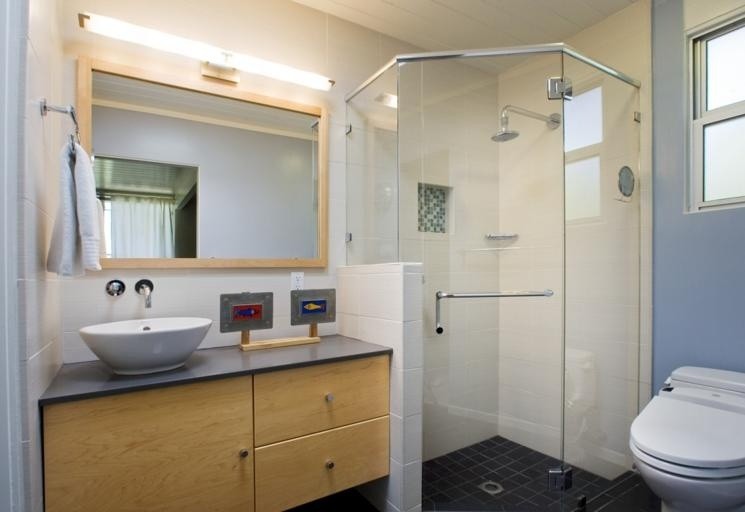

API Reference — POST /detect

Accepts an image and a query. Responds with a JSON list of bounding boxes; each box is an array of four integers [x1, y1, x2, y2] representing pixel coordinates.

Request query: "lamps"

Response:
[[73, 9, 337, 95]]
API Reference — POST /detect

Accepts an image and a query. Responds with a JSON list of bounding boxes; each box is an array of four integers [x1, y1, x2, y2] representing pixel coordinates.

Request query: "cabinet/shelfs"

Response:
[[251, 351, 396, 512], [41, 372, 254, 510]]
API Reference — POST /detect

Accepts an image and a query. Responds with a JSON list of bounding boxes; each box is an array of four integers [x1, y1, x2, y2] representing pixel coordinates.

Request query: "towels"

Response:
[[41, 137, 104, 280]]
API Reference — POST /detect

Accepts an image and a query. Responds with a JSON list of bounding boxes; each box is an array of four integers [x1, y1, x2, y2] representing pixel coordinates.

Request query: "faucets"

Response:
[[136, 279, 154, 308]]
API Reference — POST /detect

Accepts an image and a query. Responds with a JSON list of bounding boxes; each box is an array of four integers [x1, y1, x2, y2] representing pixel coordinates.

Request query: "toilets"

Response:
[[628, 365, 745, 512]]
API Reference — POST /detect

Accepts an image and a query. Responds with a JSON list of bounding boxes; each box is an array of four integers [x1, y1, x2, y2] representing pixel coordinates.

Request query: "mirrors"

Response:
[[69, 49, 335, 276]]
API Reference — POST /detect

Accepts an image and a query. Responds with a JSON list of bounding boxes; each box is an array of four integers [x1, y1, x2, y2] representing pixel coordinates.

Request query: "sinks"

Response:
[[79, 317, 213, 375]]
